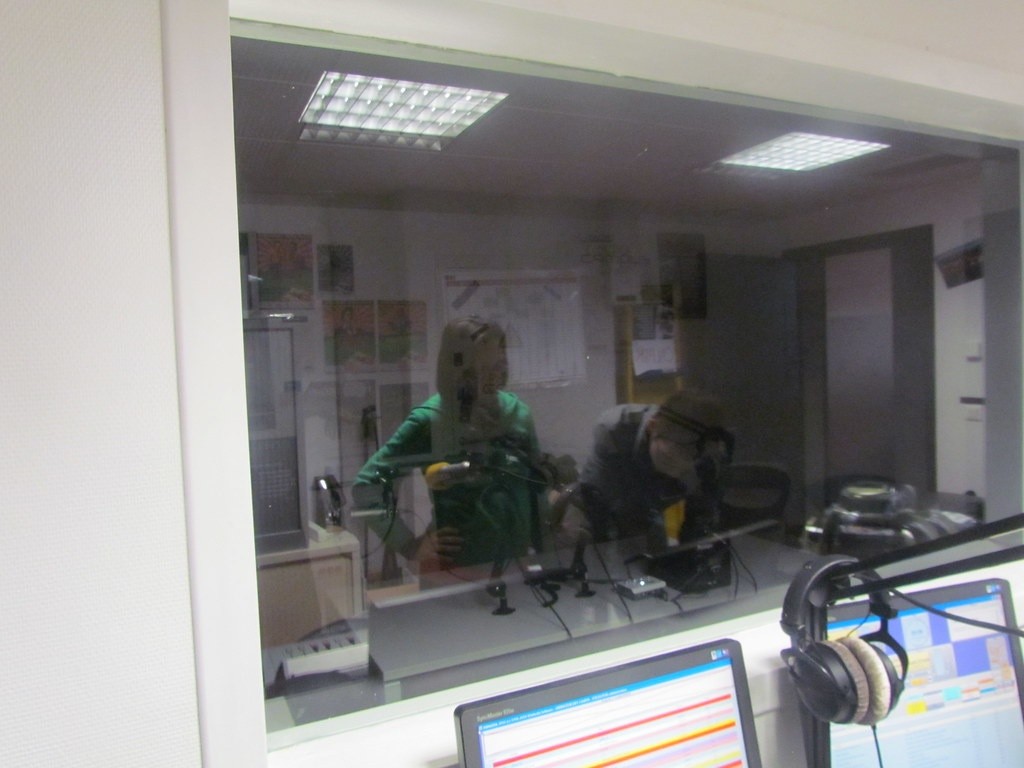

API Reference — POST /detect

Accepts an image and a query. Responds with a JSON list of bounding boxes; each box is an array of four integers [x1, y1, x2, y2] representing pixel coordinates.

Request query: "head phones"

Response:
[[780, 554, 908, 726]]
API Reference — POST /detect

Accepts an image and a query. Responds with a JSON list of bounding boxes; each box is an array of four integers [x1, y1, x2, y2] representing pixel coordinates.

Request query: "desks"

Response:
[[361, 528, 818, 682]]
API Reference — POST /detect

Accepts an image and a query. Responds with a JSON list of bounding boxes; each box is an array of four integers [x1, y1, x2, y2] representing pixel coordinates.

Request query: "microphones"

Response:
[[426, 462, 484, 490]]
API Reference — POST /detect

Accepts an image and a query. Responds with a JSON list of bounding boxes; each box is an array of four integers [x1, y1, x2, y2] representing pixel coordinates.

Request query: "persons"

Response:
[[561, 404, 724, 551], [351, 319, 555, 595]]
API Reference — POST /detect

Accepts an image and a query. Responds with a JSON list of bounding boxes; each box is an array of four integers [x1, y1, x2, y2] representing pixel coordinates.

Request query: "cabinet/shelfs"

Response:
[[259, 521, 364, 647]]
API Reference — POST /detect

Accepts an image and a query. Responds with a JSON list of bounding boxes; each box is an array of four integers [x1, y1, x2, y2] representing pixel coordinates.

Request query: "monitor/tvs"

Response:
[[453, 638, 763, 768], [813, 576, 1024, 768]]
